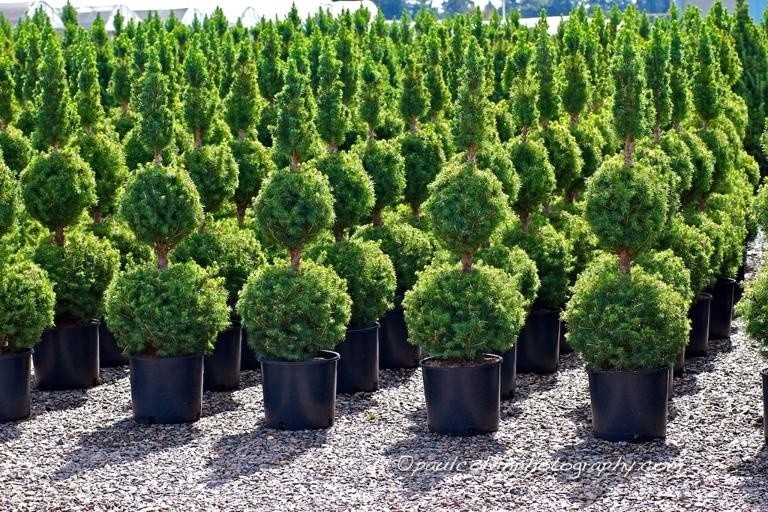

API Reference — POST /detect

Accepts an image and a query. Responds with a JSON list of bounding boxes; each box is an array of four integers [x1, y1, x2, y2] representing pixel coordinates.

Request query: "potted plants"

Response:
[[0, 0, 768, 445]]
